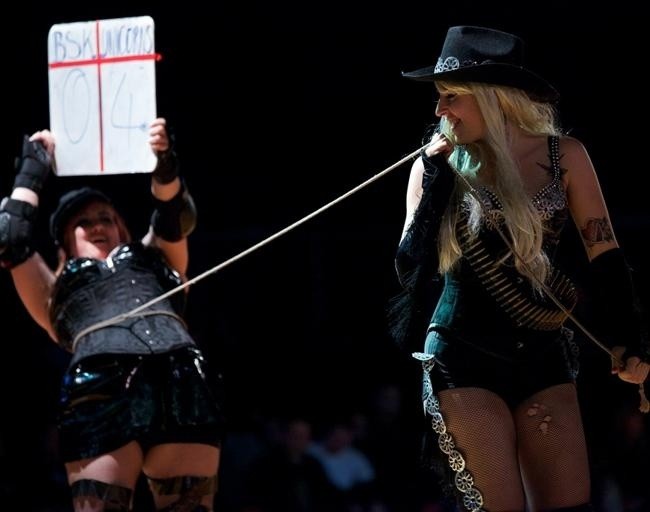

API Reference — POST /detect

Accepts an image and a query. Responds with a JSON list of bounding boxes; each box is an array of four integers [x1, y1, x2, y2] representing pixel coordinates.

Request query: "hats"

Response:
[[49, 187, 111, 244], [403, 26, 560, 103]]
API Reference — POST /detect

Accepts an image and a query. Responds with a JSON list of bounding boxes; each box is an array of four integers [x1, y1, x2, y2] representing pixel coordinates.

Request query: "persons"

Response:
[[395, 26, 649, 512], [1, 118, 222, 512], [247, 388, 441, 511]]
